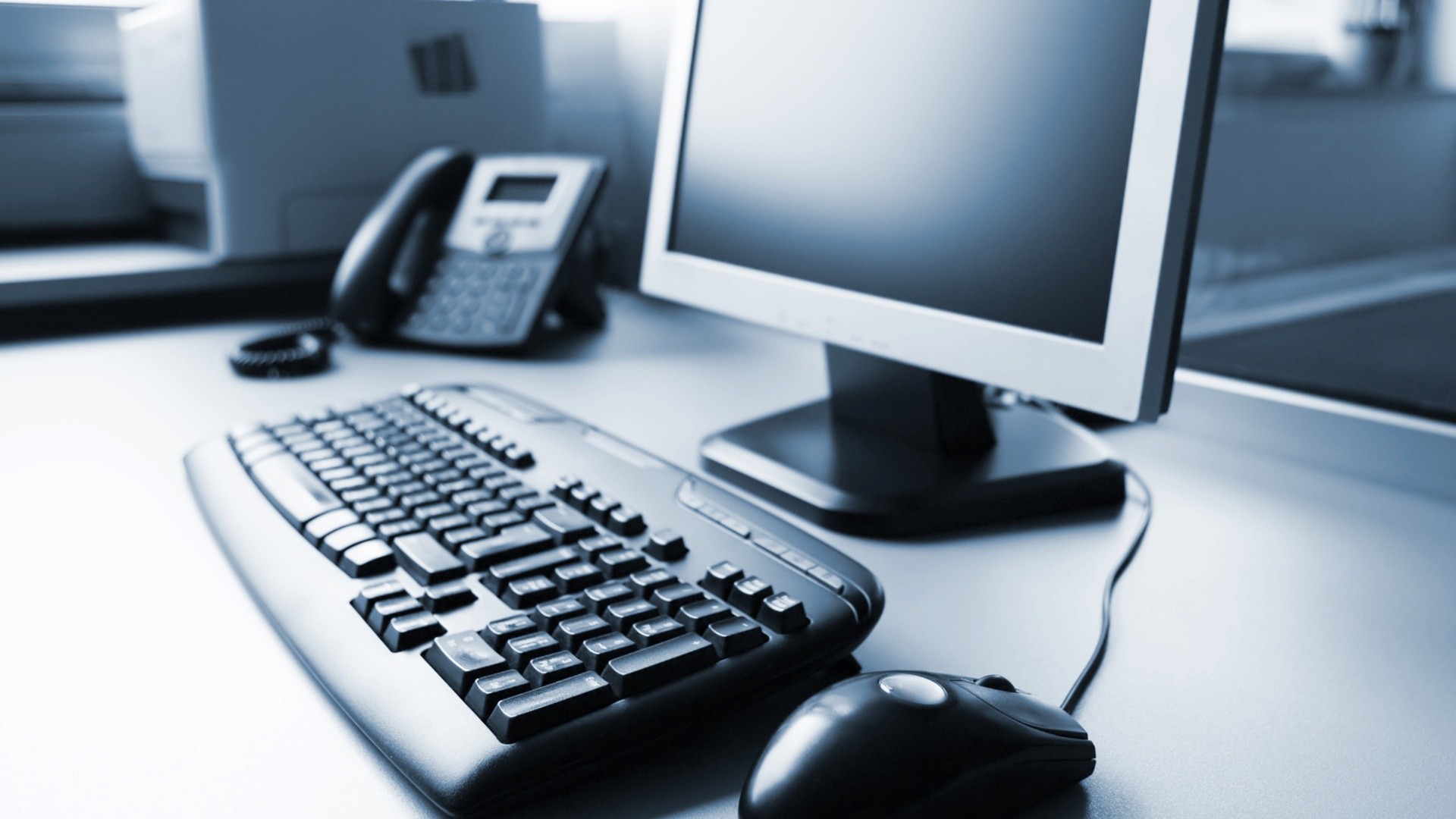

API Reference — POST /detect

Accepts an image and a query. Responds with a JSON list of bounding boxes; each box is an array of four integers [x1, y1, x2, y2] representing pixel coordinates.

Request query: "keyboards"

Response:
[[180, 381, 885, 819]]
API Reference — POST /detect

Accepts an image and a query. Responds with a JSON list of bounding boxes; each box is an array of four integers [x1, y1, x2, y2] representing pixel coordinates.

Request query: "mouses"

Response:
[[733, 671, 1099, 819]]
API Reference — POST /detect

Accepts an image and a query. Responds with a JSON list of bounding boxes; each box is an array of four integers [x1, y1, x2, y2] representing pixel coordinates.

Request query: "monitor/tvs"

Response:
[[634, 2, 1232, 541]]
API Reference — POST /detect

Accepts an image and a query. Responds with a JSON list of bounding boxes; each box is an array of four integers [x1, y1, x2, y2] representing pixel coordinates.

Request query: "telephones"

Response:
[[226, 143, 610, 379]]
